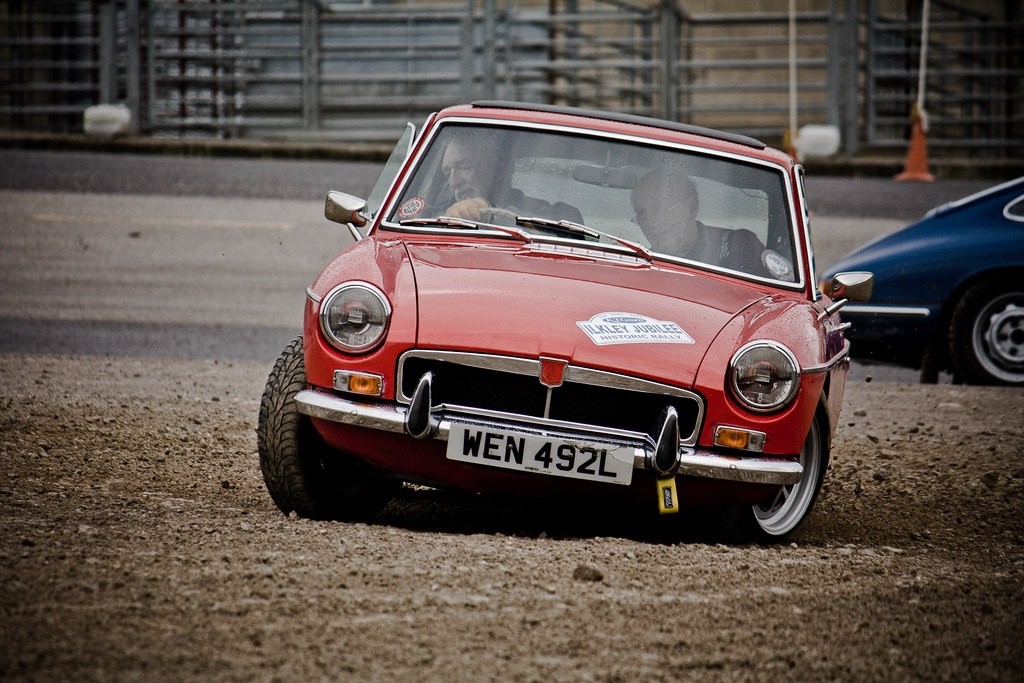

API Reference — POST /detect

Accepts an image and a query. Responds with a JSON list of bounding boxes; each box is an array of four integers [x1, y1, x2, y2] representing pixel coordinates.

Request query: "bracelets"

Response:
[[489, 202, 497, 224]]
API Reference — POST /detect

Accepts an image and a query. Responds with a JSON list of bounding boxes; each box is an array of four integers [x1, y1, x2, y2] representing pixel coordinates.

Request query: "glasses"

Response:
[[630, 198, 689, 225]]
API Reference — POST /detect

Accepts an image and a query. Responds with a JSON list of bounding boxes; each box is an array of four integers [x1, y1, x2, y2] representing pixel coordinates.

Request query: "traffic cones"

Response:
[[893, 106, 940, 182]]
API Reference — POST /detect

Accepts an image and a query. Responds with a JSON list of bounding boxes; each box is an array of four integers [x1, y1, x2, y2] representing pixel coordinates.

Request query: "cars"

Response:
[[257, 100, 873, 546], [817, 177, 1024, 388]]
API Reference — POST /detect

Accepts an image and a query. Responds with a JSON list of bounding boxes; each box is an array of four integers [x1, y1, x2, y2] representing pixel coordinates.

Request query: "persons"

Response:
[[432, 130, 586, 241], [629, 168, 773, 279]]
